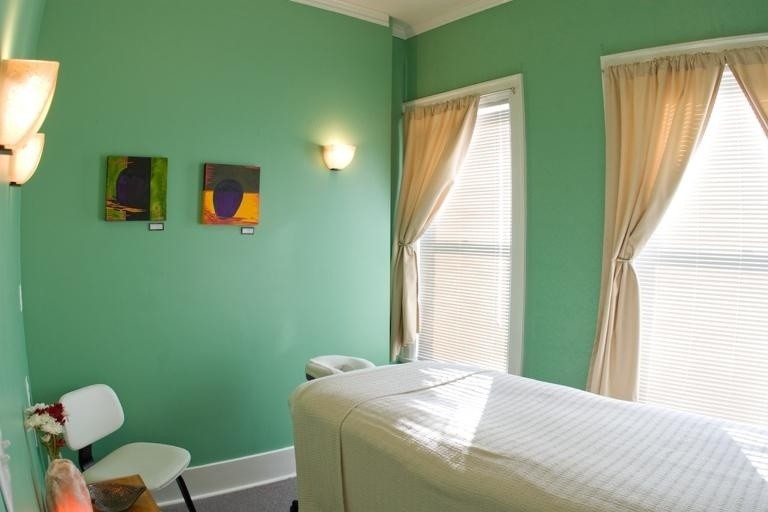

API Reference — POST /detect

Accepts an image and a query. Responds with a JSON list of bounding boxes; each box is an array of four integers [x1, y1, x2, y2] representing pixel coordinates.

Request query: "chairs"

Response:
[[56, 383, 196, 511]]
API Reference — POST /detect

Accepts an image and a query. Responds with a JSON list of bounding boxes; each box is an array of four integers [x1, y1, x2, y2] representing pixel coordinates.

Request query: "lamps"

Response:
[[321, 143, 356, 170], [0, 57, 61, 188]]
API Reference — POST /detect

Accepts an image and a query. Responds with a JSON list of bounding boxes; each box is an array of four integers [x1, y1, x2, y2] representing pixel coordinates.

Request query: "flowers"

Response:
[[25, 400, 68, 464]]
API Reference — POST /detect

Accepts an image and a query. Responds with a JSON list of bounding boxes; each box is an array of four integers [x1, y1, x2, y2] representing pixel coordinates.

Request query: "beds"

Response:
[[290, 360, 768, 511]]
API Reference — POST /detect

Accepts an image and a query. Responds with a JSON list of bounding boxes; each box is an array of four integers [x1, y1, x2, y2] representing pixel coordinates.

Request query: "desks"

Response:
[[81, 474, 161, 512]]
